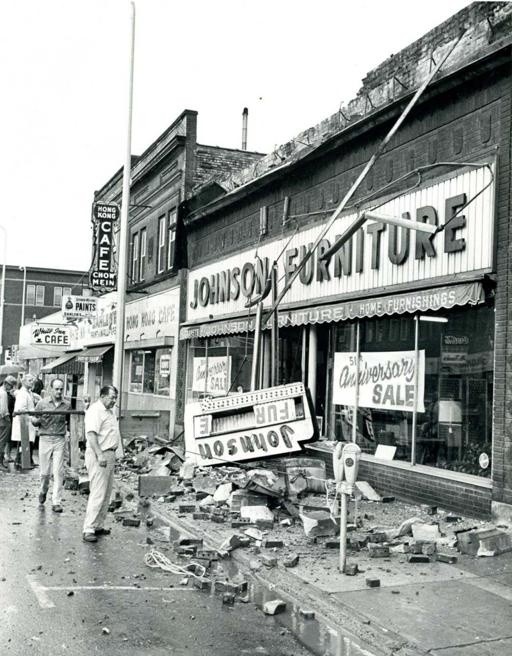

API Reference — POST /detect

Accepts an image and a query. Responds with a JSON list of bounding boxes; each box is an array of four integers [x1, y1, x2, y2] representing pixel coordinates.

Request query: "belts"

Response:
[[107, 448, 116, 451]]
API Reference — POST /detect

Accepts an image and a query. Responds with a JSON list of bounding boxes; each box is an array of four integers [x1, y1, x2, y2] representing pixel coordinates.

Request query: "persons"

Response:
[[1, 373, 45, 473], [29, 378, 73, 513], [79, 384, 121, 543]]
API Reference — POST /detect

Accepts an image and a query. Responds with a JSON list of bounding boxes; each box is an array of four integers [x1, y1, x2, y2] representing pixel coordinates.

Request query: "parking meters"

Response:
[[330, 436, 364, 574]]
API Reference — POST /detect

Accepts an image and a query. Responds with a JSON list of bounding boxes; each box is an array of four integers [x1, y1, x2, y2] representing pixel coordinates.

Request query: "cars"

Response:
[[336, 394, 446, 454]]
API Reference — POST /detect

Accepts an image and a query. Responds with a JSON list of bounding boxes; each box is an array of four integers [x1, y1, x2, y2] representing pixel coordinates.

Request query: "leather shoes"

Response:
[[52, 505, 62, 512], [84, 533, 97, 542], [95, 529, 110, 535], [39, 488, 48, 503]]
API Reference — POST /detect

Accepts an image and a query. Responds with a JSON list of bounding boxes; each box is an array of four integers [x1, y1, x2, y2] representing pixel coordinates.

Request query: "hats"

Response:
[[5, 375, 16, 386]]
